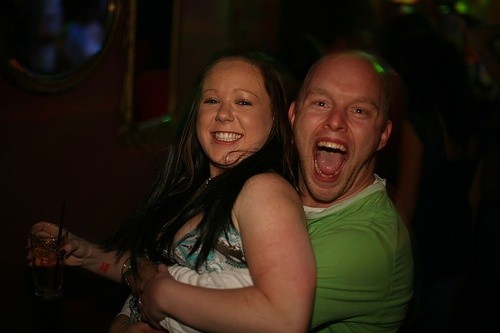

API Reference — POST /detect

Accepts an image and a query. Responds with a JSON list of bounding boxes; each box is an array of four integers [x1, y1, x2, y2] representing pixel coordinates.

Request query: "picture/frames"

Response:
[[0, 0, 125, 96]]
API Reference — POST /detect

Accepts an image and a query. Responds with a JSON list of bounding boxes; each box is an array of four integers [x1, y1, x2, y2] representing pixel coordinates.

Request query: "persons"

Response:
[[24, 48, 415, 333]]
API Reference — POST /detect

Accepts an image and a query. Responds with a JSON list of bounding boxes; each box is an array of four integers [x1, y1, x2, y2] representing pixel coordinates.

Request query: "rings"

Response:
[[138, 298, 142, 305]]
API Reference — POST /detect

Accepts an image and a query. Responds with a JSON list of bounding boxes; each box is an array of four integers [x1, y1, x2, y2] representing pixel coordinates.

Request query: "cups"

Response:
[[25, 226, 68, 278]]
